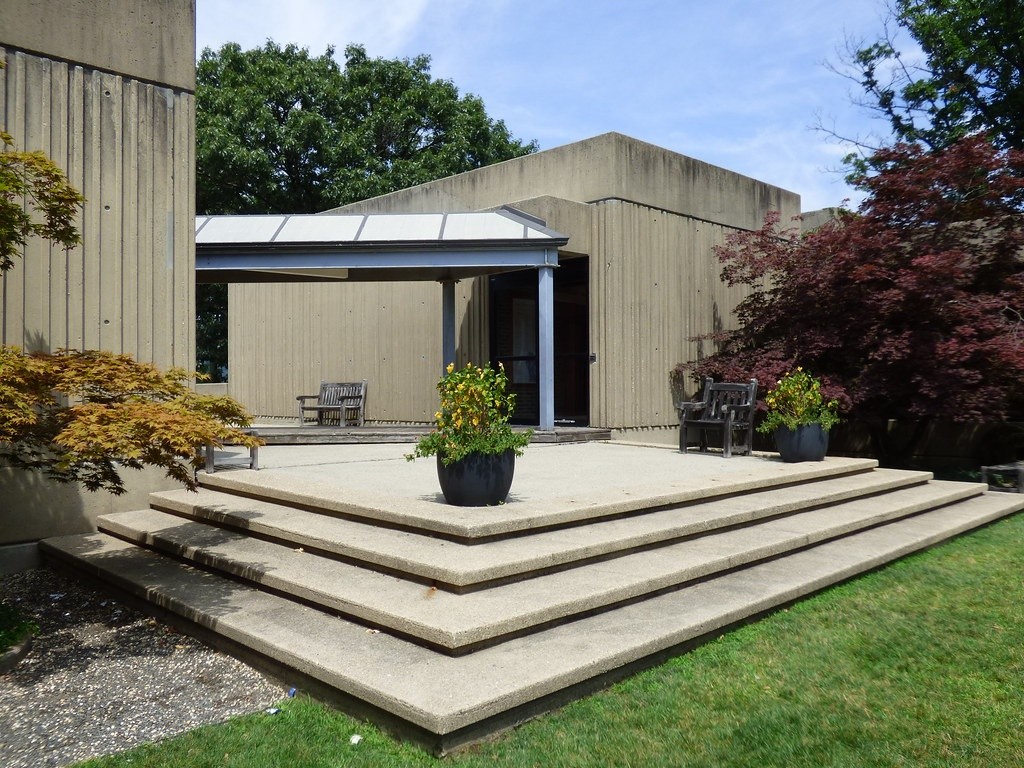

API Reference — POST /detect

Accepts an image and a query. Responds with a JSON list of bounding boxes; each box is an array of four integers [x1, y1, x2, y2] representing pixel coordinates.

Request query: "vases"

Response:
[[773, 422, 829, 462], [436, 447, 514, 506]]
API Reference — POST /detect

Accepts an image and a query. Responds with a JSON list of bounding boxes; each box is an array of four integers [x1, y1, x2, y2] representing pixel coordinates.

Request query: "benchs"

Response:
[[981, 460, 1024, 493], [195, 430, 259, 474], [296, 379, 368, 428]]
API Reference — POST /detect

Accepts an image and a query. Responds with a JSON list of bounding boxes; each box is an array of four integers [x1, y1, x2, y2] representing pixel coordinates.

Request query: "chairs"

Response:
[[680, 377, 759, 457]]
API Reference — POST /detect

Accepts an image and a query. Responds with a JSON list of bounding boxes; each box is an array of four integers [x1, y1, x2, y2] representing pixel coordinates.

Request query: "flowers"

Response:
[[401, 360, 534, 470], [755, 367, 840, 434]]
